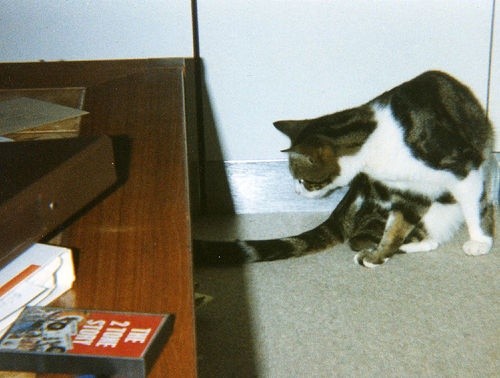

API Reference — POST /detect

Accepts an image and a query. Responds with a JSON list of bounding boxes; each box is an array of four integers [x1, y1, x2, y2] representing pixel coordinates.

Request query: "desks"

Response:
[[0, 57, 200, 378]]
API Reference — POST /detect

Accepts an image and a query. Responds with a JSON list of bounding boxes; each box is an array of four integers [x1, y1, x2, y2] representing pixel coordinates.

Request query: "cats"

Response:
[[191, 69, 498, 271]]
